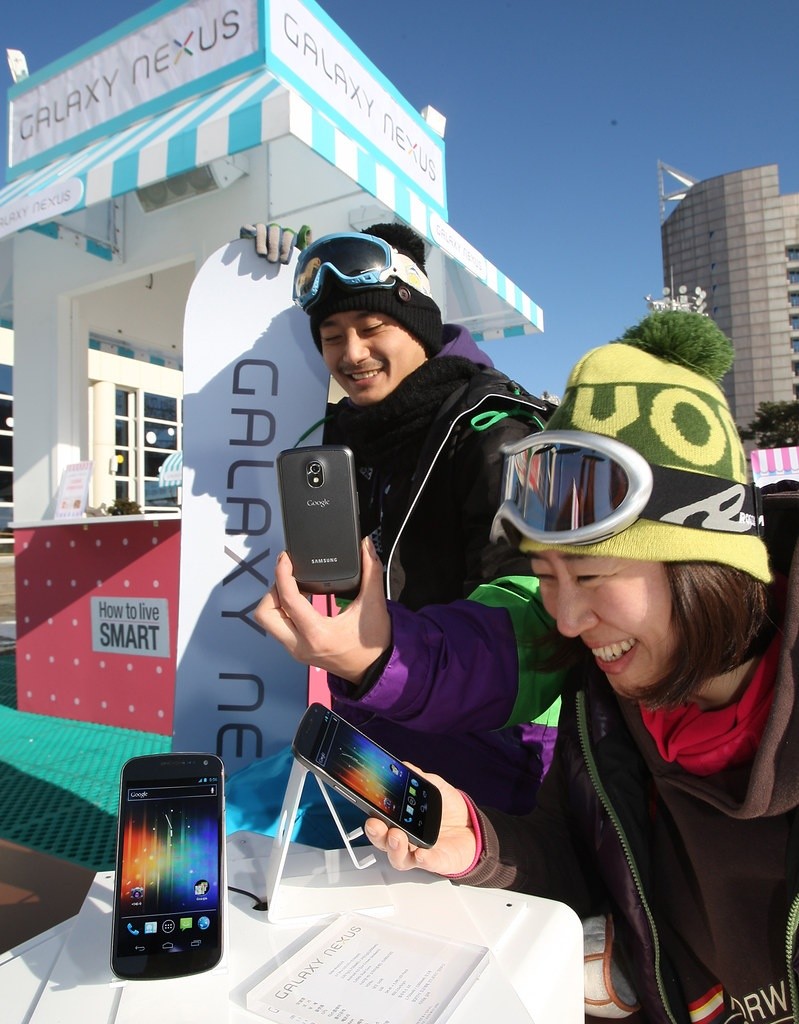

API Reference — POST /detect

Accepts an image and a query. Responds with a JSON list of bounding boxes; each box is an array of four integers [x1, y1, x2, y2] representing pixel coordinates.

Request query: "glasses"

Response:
[[293, 230, 398, 315], [487, 428, 655, 550]]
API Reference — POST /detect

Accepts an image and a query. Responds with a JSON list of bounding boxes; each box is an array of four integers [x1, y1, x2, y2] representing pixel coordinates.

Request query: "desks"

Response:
[[0, 830, 586, 1024]]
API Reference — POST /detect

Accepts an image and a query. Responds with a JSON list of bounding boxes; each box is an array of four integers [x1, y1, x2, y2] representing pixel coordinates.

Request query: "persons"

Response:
[[362, 309, 799, 1024], [256, 223, 582, 820]]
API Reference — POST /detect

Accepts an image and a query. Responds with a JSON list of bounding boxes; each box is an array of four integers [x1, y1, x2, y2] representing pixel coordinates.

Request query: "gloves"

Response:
[[239, 222, 313, 265]]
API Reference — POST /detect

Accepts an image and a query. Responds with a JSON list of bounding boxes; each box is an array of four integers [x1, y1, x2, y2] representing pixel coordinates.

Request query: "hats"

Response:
[[519, 308, 775, 583], [309, 223, 442, 360]]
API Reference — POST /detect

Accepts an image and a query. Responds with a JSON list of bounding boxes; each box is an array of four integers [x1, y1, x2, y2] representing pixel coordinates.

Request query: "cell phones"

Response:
[[110, 751, 228, 980], [277, 445, 363, 596], [291, 702, 442, 849]]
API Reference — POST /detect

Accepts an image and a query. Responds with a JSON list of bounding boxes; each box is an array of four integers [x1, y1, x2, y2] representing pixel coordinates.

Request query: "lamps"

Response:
[[134, 152, 250, 214]]
